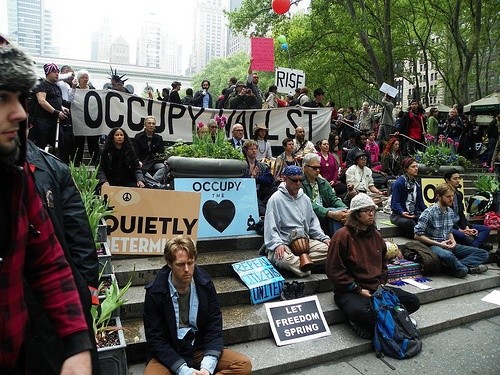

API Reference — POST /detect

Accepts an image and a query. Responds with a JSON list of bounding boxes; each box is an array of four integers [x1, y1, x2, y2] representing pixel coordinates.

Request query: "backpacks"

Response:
[[370, 285, 422, 360], [402, 241, 439, 266], [291, 95, 304, 106]]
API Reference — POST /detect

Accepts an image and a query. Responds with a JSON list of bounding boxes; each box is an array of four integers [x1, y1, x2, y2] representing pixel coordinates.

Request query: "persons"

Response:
[[264, 165, 331, 277], [24, 139, 100, 375], [0, 35, 94, 375], [326, 193, 420, 338], [414, 184, 489, 277], [27, 58, 425, 215], [390, 158, 427, 231], [445, 168, 493, 251], [143, 235, 252, 375], [302, 152, 349, 235], [426, 104, 500, 187]]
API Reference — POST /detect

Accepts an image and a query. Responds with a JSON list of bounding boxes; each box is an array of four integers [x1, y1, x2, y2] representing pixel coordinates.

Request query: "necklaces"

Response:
[[257, 141, 266, 154]]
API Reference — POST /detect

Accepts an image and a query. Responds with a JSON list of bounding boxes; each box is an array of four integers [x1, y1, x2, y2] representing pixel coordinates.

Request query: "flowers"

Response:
[[164, 115, 245, 160]]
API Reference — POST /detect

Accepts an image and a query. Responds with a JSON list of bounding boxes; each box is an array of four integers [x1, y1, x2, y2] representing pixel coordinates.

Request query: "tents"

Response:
[[425, 91, 500, 125]]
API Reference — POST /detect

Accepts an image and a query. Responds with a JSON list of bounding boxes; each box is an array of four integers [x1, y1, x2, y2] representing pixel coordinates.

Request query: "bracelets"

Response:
[[53, 110, 60, 117]]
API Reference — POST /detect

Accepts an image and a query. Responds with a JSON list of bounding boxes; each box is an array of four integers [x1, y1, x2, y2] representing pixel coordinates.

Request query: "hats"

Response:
[[0, 35, 36, 94], [253, 122, 270, 134], [348, 192, 378, 214], [43, 63, 60, 75], [355, 152, 367, 161], [313, 89, 325, 95], [77, 69, 89, 80], [234, 81, 246, 87]]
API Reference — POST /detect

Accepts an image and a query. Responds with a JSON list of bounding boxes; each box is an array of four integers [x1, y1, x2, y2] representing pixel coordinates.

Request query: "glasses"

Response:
[[358, 209, 376, 215], [232, 129, 244, 132], [252, 77, 259, 78], [305, 164, 322, 171], [286, 175, 305, 183], [358, 156, 367, 160]]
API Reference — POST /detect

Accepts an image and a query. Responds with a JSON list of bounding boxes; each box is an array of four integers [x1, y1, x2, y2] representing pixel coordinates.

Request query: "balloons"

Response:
[[272, 0, 290, 14], [278, 35, 287, 50]]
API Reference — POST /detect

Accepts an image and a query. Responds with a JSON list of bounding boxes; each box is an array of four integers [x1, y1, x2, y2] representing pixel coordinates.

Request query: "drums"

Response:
[[289, 236, 315, 271]]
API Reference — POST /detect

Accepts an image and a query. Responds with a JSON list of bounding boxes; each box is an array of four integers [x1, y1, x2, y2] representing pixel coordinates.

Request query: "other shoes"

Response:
[[157, 184, 170, 189], [479, 243, 493, 251], [472, 264, 488, 274]]
